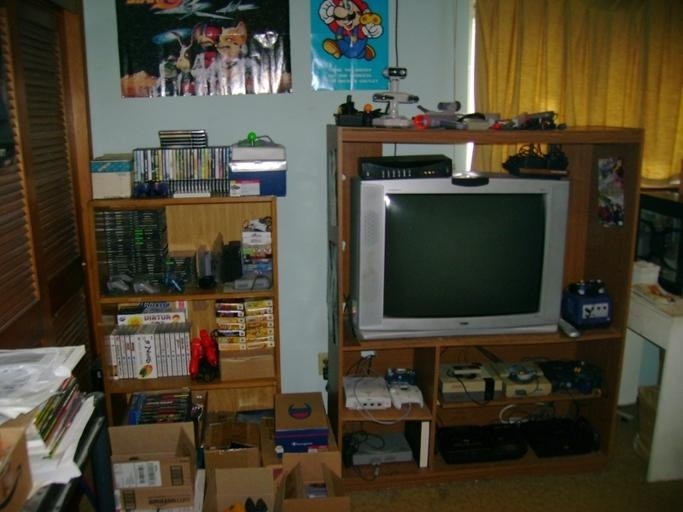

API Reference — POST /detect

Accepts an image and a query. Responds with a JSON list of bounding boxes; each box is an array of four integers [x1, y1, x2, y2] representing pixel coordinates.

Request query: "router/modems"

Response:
[[345, 363, 599, 472]]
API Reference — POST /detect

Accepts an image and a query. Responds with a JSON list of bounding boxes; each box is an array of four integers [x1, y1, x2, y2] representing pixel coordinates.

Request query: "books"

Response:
[[25, 372, 104, 484]]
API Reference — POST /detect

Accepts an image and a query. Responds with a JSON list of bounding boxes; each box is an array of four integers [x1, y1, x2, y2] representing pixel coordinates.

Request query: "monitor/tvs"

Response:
[[349, 172, 569, 340]]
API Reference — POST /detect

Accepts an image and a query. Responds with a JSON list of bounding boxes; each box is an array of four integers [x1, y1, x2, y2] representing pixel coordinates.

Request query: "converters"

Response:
[[323, 359, 328, 379]]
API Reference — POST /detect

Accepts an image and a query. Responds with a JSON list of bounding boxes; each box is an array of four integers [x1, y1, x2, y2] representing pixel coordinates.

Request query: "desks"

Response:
[[609, 273, 680, 484]]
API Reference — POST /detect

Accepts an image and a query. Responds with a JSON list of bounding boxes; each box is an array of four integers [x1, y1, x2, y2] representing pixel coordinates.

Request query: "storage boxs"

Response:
[[229, 159, 285, 195], [215, 346, 275, 381], [89, 151, 132, 197], [1, 406, 40, 512], [105, 388, 351, 512]]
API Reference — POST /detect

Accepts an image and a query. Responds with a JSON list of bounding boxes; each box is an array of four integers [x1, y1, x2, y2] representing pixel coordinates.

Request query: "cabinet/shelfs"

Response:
[[323, 121, 646, 491], [88, 197, 285, 431]]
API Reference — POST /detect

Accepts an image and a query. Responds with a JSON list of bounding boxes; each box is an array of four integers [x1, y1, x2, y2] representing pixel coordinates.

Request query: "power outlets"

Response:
[[319, 351, 330, 375]]
